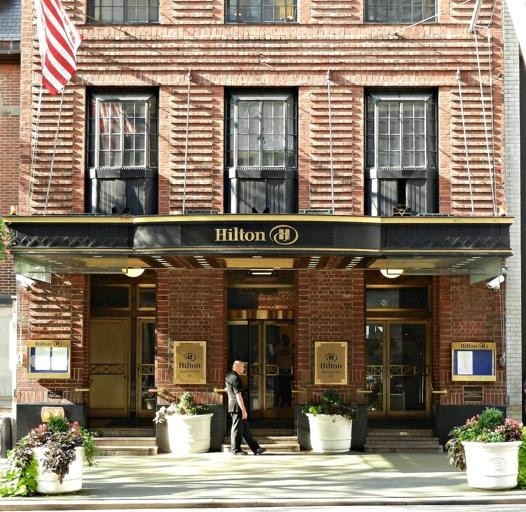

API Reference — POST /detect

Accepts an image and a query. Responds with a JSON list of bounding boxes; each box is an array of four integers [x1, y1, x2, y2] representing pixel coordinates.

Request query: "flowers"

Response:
[[0, 413, 99, 496], [446, 407, 522, 470]]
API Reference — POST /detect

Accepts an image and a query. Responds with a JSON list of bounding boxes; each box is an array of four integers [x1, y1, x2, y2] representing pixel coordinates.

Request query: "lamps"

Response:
[[380, 269, 403, 278], [122, 269, 144, 277]]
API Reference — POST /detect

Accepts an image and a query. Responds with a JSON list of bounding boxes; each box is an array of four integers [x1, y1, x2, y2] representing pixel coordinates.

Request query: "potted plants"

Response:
[[153, 394, 214, 453], [302, 388, 363, 453]]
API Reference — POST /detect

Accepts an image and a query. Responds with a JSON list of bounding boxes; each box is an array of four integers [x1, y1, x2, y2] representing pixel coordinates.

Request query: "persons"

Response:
[[225, 360, 267, 456], [275, 334, 295, 408]]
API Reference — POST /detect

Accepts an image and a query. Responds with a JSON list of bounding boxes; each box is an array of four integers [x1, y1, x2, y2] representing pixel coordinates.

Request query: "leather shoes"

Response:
[[233, 448, 268, 456]]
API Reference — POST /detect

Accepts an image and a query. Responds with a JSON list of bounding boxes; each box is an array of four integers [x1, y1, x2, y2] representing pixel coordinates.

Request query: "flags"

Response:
[[35, 0, 83, 96], [92, 98, 135, 148]]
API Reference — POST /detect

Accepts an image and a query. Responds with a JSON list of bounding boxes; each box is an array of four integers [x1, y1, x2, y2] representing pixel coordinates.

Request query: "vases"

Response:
[[462, 442, 521, 489], [31, 447, 82, 494]]
